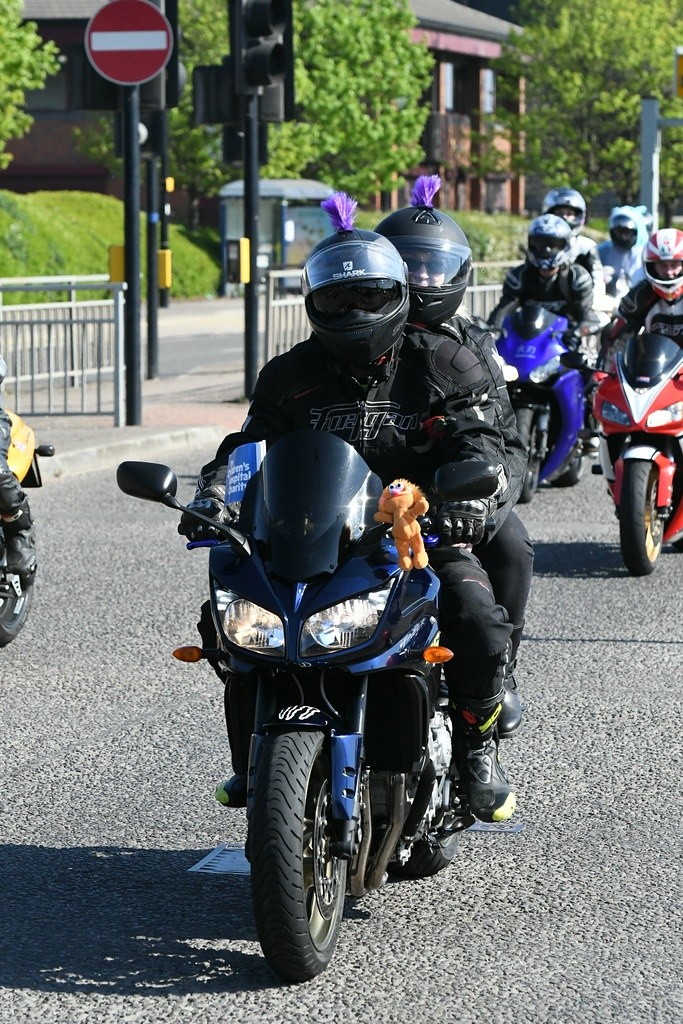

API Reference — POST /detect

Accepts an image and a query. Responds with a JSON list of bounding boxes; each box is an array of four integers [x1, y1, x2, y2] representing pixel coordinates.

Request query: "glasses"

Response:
[[402, 257, 450, 274]]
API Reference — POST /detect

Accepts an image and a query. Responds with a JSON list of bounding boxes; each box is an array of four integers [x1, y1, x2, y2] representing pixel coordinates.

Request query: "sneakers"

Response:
[[452, 720, 515, 821], [216, 772, 248, 807]]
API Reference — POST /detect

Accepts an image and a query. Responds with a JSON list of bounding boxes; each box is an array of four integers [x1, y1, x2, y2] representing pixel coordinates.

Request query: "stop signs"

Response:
[[84, 0, 170, 85]]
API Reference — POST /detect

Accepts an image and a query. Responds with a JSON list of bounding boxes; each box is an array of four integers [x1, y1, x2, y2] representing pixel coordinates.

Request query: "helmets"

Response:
[[300, 229, 409, 363], [609, 206, 638, 249], [373, 204, 473, 326], [642, 228, 683, 306], [541, 187, 586, 236], [527, 214, 573, 272]]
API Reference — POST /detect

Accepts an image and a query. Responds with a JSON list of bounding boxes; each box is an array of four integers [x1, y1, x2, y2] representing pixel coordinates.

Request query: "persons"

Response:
[[579, 228, 683, 372], [598, 205, 650, 290], [372, 207, 535, 739], [178, 227, 514, 823], [486, 214, 600, 459], [541, 187, 606, 312], [0, 354, 38, 590]]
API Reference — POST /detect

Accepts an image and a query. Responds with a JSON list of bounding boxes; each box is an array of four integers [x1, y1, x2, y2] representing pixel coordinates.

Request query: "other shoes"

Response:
[[5, 522, 36, 585]]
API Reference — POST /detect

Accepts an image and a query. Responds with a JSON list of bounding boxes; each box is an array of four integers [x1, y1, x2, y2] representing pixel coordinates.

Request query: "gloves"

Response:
[[177, 485, 242, 542], [440, 464, 507, 543]]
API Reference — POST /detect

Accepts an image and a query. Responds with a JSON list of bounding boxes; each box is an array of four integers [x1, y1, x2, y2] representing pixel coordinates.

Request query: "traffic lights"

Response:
[[227, 0, 293, 94]]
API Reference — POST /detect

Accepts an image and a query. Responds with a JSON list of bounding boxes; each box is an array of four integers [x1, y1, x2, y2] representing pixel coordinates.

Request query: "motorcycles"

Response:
[[116, 428, 478, 982], [0, 407, 55, 645], [559, 329, 683, 577], [466, 298, 612, 504]]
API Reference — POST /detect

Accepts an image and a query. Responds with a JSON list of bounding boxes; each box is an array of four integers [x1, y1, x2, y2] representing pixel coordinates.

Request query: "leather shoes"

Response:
[[496, 674, 524, 738]]
